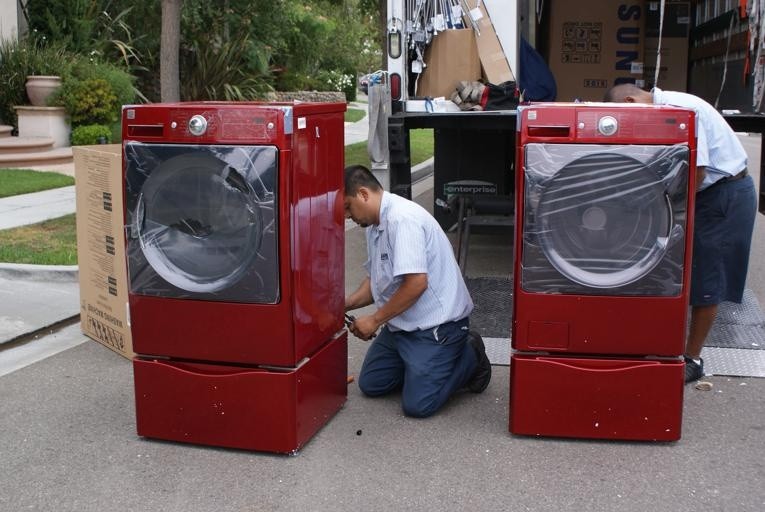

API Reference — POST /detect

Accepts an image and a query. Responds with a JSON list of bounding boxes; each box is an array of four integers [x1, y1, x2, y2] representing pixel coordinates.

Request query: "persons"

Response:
[[603, 82, 757, 384], [343, 162, 493, 417]]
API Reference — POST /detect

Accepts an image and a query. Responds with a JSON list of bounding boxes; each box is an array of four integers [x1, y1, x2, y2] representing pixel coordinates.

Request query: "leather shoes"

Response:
[[466, 330, 492, 394]]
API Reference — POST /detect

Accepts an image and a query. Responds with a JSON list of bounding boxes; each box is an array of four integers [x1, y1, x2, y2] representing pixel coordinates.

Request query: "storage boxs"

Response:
[[72, 144, 135, 364], [415, 25, 482, 103], [534, 0, 689, 112]]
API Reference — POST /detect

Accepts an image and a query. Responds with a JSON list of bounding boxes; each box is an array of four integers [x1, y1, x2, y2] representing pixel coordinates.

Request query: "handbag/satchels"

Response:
[[450, 78, 521, 111]]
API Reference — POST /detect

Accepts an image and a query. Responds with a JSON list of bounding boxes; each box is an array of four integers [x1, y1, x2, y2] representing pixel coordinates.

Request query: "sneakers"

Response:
[[683, 354, 703, 383]]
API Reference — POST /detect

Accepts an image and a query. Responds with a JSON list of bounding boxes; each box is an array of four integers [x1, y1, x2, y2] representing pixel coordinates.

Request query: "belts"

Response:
[[718, 167, 749, 183]]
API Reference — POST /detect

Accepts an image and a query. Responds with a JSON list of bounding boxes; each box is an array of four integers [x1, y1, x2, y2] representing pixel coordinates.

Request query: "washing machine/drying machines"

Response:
[[121, 103, 347, 453], [509, 104, 695, 442]]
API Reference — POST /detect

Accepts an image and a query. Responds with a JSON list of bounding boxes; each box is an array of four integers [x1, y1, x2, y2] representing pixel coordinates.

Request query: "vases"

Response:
[[25, 75, 61, 108]]
